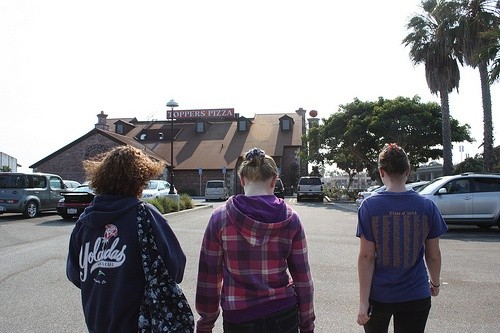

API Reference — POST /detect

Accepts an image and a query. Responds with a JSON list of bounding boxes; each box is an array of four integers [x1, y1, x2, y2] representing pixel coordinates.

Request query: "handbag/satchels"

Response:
[[138, 277, 194, 333]]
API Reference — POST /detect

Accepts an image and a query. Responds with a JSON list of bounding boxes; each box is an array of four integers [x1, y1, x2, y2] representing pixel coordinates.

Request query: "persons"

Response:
[[355, 143, 448, 333], [67, 144, 195, 333], [195, 148, 316, 333]]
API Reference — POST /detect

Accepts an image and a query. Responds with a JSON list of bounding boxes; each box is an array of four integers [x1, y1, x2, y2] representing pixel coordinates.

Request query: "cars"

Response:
[[36, 179, 98, 220], [355, 172, 500, 230], [138, 180, 177, 204], [274, 177, 286, 202]]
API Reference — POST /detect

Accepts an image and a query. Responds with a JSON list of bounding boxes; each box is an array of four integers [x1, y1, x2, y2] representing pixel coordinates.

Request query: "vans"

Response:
[[205, 180, 229, 201], [0, 172, 69, 218]]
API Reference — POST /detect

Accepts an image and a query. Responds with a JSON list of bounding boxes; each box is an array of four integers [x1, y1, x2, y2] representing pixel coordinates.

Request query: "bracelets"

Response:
[[430, 279, 440, 287]]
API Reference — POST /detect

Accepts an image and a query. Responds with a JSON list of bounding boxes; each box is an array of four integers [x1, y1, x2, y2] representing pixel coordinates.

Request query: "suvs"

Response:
[[296, 176, 326, 202]]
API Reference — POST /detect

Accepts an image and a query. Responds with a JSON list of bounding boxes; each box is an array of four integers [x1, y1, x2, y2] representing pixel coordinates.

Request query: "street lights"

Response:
[[165, 99, 179, 199]]
